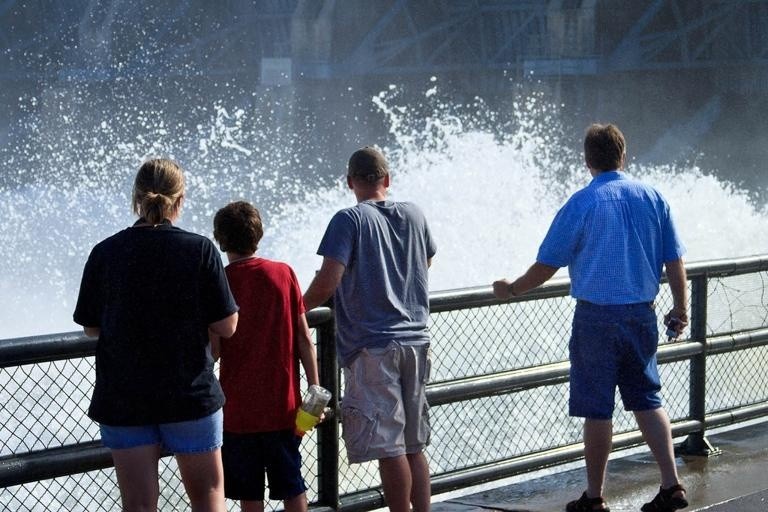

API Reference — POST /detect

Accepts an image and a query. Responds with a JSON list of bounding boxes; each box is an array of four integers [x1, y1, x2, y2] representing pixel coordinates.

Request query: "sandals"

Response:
[[563, 493, 610, 512], [639, 486, 688, 511]]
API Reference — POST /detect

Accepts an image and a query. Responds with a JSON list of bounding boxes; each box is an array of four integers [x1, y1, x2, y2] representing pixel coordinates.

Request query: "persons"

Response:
[[297, 143, 439, 512], [202, 200, 325, 511], [72, 160, 242, 511], [490, 120, 693, 511]]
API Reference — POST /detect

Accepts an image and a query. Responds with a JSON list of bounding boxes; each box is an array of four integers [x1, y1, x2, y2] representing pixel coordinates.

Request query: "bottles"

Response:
[[295, 384, 332, 437]]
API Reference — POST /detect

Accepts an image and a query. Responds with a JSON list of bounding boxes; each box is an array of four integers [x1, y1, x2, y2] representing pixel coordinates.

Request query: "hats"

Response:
[[348, 146, 388, 181]]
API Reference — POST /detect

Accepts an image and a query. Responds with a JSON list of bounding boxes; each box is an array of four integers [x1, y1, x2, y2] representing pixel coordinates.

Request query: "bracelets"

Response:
[[507, 282, 519, 300]]
